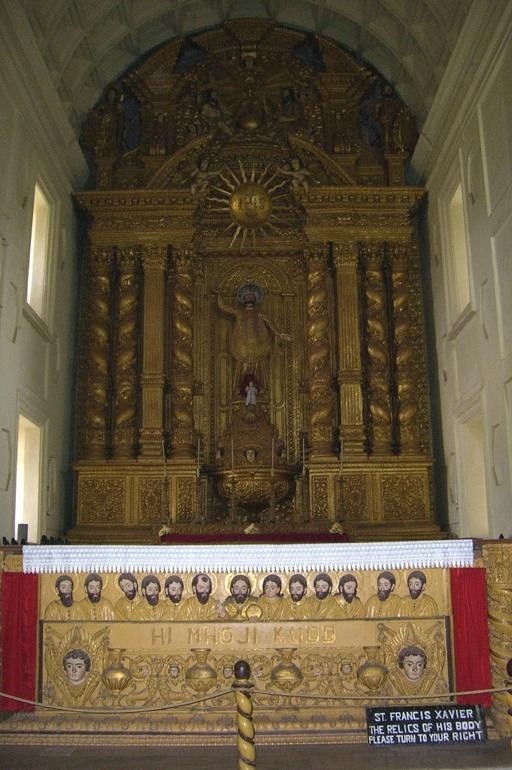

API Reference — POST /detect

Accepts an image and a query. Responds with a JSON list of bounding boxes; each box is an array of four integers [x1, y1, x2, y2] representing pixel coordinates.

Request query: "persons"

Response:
[[245, 379, 258, 404], [274, 155, 313, 199], [216, 283, 295, 398], [263, 86, 307, 141], [190, 156, 222, 192], [115, 572, 365, 620], [90, 87, 123, 161], [48, 572, 114, 619], [201, 86, 229, 141], [387, 646, 438, 708], [54, 649, 102, 702], [366, 572, 439, 618], [372, 79, 406, 155]]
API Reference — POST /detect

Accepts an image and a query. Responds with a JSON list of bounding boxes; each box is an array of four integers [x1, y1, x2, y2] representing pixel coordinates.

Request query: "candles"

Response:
[[160, 437, 345, 478]]
